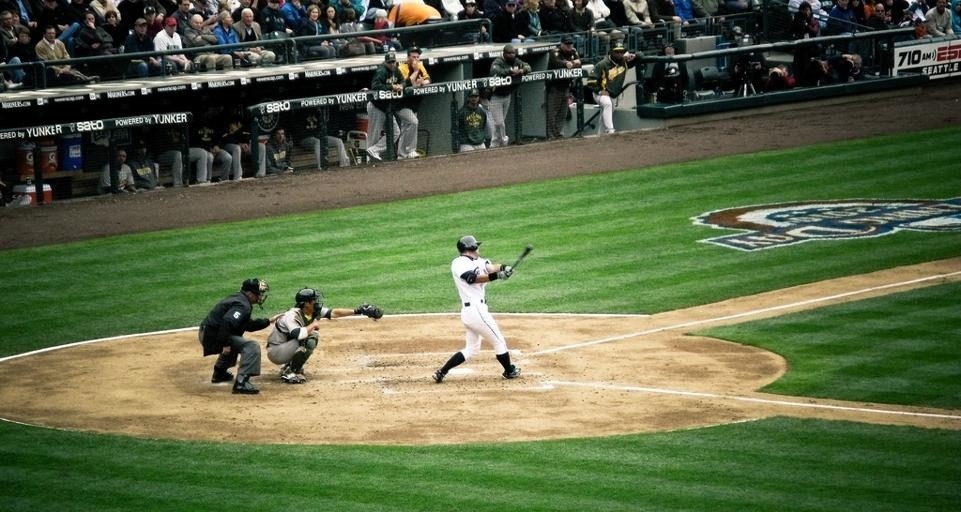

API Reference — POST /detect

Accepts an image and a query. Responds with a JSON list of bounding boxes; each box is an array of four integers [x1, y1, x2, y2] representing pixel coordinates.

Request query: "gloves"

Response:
[[497, 265, 512, 279]]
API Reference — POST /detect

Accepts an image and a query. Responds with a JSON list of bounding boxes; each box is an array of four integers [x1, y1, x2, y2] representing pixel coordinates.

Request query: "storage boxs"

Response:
[[12, 182, 52, 206]]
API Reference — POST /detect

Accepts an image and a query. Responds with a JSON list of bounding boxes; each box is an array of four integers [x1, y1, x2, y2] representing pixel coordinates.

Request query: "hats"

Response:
[[465, 0, 477, 7], [468, 89, 480, 96], [407, 46, 422, 55], [561, 35, 575, 44], [507, 0, 517, 5], [385, 53, 396, 63], [732, 26, 742, 34], [134, 6, 177, 26]]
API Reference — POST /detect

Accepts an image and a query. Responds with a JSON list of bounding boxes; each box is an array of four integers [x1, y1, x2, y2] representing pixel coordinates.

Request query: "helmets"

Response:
[[457, 235, 482, 251], [610, 42, 625, 52], [242, 279, 269, 310], [295, 289, 325, 315]]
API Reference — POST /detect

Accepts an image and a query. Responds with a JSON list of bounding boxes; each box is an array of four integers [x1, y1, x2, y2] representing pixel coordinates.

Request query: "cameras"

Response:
[[737, 54, 752, 67], [771, 71, 781, 81], [843, 61, 852, 70]]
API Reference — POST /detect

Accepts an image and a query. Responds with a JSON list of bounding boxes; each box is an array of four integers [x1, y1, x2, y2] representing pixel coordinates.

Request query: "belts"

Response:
[[464, 299, 487, 306]]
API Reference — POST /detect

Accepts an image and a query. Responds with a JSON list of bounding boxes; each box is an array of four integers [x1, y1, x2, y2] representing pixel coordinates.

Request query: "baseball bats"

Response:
[[510, 245, 533, 272]]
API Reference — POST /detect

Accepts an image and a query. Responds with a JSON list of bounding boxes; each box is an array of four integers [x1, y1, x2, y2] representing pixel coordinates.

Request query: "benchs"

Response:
[[69, 141, 353, 198]]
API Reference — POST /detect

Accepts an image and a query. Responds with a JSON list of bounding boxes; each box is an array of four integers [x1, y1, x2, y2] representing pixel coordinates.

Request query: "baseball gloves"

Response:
[[354, 304, 383, 319]]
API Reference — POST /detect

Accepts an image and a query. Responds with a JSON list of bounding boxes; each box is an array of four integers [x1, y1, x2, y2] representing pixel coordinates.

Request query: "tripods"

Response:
[[736, 67, 756, 97]]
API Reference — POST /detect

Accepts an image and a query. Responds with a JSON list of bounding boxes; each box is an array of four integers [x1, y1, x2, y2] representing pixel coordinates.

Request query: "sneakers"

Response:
[[212, 365, 306, 394], [407, 151, 419, 158], [501, 365, 521, 380], [432, 369, 445, 382], [366, 147, 382, 162]]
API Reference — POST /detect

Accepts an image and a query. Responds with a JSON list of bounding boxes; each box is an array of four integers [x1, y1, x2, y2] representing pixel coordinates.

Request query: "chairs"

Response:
[[261, 10, 390, 59], [644, 61, 729, 105]]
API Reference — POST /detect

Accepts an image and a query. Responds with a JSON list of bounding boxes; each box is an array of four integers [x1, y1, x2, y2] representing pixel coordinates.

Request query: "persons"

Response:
[[432, 233, 522, 383], [1, 0, 637, 206], [266, 287, 370, 384], [623, 0, 961, 103], [197, 278, 283, 395]]
